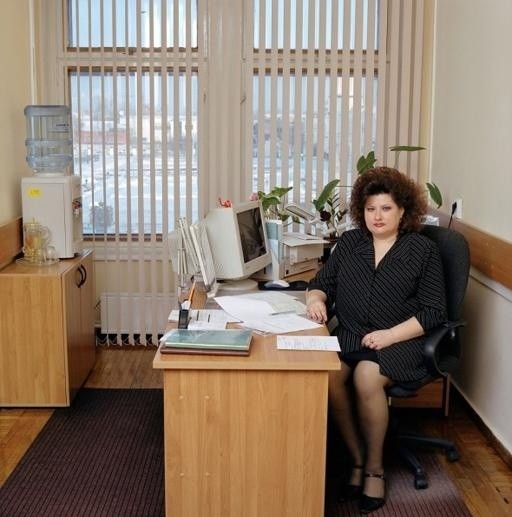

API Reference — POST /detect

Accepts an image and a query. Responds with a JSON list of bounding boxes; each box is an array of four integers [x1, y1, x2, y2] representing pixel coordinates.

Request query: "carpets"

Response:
[[1, 387, 476, 517]]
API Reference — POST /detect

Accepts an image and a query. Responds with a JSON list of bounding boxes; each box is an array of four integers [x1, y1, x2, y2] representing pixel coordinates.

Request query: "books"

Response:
[[267, 221, 285, 264], [163, 329, 255, 351], [190, 222, 216, 285], [160, 346, 250, 357]]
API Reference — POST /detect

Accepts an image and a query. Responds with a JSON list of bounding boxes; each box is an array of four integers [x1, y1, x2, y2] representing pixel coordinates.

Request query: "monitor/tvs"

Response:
[[203, 200, 272, 291]]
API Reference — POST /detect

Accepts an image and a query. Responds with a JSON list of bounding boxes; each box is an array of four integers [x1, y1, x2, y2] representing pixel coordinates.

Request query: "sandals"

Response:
[[342, 464, 363, 502], [358, 462, 385, 513]]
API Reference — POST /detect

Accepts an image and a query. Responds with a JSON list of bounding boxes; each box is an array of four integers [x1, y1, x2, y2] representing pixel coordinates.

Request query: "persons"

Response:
[[305, 166, 449, 513]]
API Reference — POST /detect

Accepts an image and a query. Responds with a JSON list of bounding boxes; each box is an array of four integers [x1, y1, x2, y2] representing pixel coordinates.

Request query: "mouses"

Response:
[[264, 280, 289, 288]]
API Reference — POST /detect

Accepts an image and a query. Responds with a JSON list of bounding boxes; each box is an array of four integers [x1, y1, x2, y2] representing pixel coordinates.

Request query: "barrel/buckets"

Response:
[[25, 105, 72, 173]]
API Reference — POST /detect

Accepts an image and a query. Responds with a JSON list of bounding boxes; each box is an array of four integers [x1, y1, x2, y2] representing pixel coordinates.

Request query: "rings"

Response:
[[369, 339, 376, 344]]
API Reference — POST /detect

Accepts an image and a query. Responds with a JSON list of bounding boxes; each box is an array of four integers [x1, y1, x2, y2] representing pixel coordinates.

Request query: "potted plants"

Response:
[[309, 187, 349, 265]]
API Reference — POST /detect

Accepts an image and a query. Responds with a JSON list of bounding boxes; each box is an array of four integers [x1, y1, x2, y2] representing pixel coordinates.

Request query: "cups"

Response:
[[46, 246, 56, 263]]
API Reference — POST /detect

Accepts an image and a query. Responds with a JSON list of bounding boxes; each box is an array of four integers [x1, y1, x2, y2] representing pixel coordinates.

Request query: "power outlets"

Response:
[[451, 197, 462, 218]]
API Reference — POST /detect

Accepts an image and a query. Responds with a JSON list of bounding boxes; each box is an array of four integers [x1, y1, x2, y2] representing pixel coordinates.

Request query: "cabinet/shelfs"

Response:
[[0, 249, 97, 408]]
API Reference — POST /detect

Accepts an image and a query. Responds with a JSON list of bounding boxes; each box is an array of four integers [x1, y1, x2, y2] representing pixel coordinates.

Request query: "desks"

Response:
[[152, 263, 344, 517]]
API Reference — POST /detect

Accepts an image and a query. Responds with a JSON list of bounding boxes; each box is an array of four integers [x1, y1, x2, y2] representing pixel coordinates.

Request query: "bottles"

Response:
[[24, 223, 49, 264]]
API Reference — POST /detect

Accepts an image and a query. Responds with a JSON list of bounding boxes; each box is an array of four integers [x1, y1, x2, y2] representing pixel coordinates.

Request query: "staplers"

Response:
[[178, 310, 190, 329]]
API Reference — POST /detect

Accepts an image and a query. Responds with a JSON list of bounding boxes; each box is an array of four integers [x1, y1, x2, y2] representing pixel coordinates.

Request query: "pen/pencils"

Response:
[[188, 279, 196, 302]]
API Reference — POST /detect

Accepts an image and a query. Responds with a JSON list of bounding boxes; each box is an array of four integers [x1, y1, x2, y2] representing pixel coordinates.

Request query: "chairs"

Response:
[[331, 224, 471, 491]]
